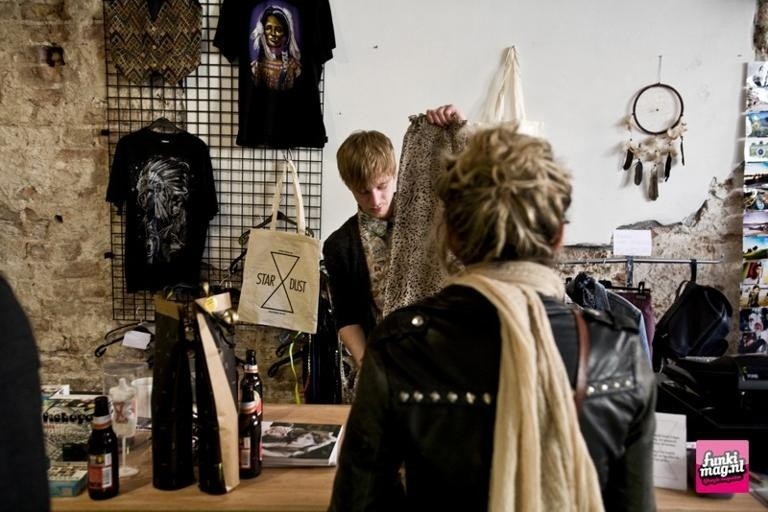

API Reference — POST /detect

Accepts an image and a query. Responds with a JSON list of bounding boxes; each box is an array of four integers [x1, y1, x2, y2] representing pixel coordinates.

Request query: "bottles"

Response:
[[238, 349, 263, 480], [87, 396, 120, 501]]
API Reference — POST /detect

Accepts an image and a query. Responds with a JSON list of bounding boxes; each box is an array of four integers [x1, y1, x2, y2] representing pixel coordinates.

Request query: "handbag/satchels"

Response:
[[652, 280, 733, 373], [238, 229, 324, 334]]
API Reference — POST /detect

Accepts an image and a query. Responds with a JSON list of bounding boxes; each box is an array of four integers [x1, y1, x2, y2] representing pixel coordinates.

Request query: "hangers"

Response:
[[118, 99, 211, 172], [239, 194, 315, 247]]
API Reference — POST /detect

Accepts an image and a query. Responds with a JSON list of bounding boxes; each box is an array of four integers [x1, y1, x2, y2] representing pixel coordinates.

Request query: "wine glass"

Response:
[[102, 361, 140, 479]]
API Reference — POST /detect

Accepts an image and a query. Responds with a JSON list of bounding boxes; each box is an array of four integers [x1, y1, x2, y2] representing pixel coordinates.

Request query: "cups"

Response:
[[131, 376, 153, 418]]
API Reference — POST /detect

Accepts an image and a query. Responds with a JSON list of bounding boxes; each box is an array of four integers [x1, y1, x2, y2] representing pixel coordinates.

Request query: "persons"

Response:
[[322, 104, 460, 404], [252, 7, 304, 89], [328, 130, 660, 512]]
